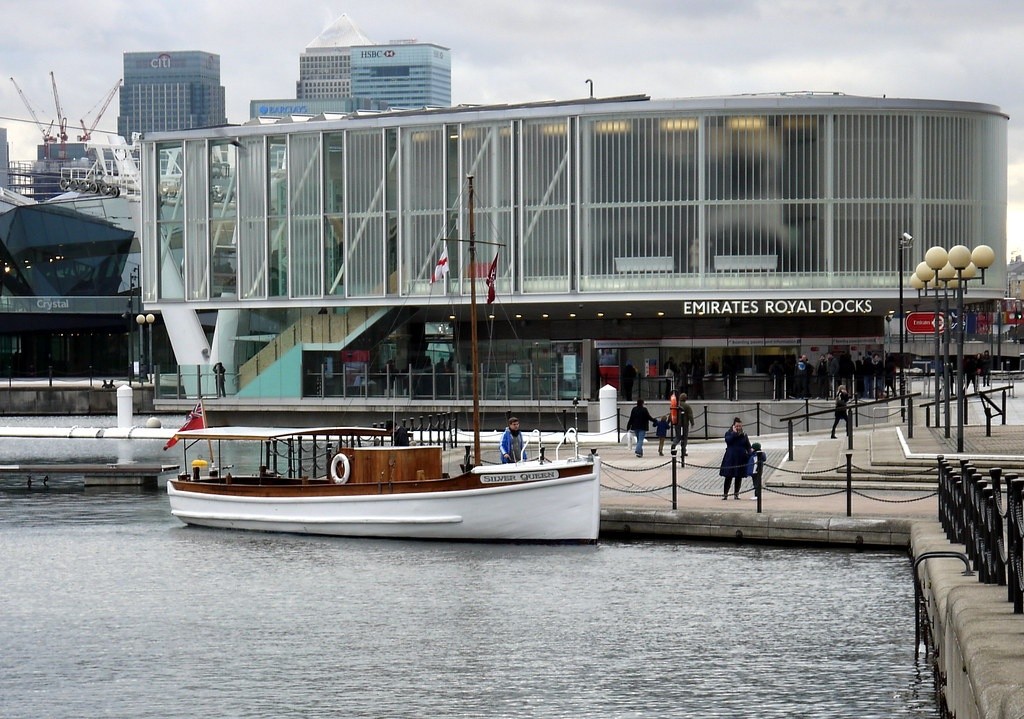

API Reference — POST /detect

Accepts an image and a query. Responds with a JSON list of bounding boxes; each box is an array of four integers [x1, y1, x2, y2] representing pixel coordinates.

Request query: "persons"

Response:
[[719, 417, 752, 500], [831, 384, 853, 438], [499, 417, 527, 464], [436, 352, 454, 375], [626, 399, 660, 458], [663, 356, 707, 400], [769, 352, 911, 400], [943, 350, 991, 396], [213, 262, 232, 273], [666, 393, 695, 456], [496, 381, 506, 400], [747, 443, 766, 501], [212, 362, 227, 398], [587, 359, 606, 401], [652, 415, 671, 456], [707, 359, 719, 374], [621, 359, 637, 401], [386, 420, 409, 446]]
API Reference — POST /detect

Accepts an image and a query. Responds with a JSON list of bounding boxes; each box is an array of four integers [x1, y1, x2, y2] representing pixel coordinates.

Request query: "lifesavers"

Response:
[[329, 452, 351, 485], [669, 394, 677, 425]]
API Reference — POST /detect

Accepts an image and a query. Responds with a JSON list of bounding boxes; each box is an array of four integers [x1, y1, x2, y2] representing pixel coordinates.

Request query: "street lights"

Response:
[[146, 314, 155, 383], [915, 260, 977, 439], [926, 245, 994, 454], [136, 315, 146, 382], [909, 272, 964, 428]]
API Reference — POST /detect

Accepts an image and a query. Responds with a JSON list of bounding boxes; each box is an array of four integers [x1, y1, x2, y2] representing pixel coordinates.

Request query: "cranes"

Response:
[[79, 78, 123, 142], [8, 77, 55, 160], [50, 70, 69, 160]]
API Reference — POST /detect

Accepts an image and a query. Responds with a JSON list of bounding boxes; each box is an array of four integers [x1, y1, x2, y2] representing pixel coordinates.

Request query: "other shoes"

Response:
[[723, 497, 727, 500], [734, 495, 739, 500], [831, 435, 837, 438], [750, 496, 758, 500]]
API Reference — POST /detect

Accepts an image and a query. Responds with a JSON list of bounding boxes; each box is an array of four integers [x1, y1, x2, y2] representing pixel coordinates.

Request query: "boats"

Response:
[[169, 174, 599, 540]]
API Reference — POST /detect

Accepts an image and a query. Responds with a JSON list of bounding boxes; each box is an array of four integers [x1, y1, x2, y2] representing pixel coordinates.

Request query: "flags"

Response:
[[163, 399, 205, 451], [429, 246, 449, 286], [485, 251, 499, 310]]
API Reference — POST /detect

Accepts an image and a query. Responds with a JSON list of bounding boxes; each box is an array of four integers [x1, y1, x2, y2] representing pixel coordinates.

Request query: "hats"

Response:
[[751, 443, 762, 450], [680, 393, 687, 401], [384, 421, 397, 429], [661, 415, 667, 421]]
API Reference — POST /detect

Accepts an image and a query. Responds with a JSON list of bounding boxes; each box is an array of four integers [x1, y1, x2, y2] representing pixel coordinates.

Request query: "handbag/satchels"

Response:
[[740, 454, 748, 470], [835, 393, 846, 407]]
